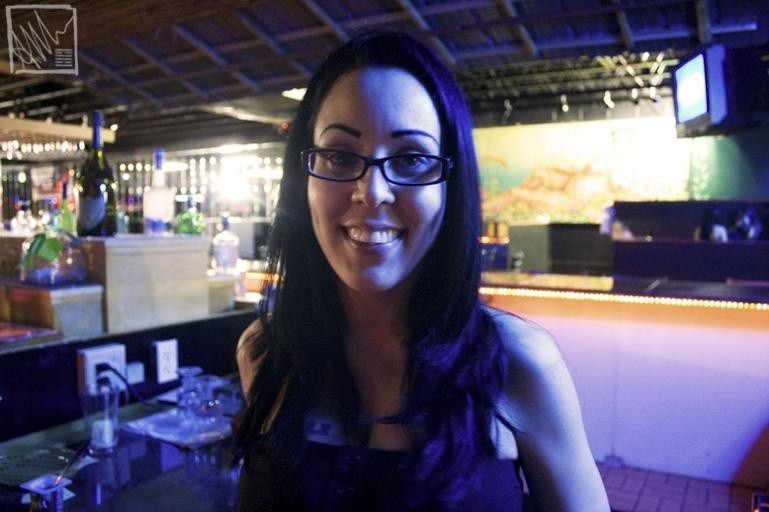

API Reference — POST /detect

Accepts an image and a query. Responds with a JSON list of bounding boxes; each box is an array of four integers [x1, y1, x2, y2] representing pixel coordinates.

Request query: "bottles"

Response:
[[77, 109, 117, 238], [143, 149, 175, 238], [176, 195, 203, 236], [58, 182, 73, 235], [210, 218, 240, 277]]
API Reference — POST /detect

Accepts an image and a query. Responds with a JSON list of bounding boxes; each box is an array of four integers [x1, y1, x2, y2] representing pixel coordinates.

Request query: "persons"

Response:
[[694, 206, 729, 243], [225, 20, 612, 511], [725, 206, 764, 241]]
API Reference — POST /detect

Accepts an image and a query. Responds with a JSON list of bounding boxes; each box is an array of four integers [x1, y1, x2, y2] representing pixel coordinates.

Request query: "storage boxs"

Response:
[[25, 232, 215, 330], [8, 273, 102, 338], [208, 273, 239, 314]]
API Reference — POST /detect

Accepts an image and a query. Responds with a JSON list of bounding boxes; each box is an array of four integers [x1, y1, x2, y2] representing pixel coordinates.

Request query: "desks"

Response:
[[1, 368, 248, 512], [478, 272, 768, 488]]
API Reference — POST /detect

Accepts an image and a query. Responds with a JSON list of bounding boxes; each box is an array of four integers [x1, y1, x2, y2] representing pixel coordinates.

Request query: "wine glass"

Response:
[[175, 365, 208, 431], [0, 130, 88, 163]]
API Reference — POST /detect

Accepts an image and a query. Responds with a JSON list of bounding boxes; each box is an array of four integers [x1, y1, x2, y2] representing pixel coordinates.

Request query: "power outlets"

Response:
[[78, 341, 126, 398]]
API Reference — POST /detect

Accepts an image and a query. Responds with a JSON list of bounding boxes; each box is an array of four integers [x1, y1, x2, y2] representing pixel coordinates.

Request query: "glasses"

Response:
[[297, 147, 454, 187]]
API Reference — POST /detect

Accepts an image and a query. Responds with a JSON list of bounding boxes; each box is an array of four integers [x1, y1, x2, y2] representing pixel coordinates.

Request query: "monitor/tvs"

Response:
[[671, 43, 728, 138]]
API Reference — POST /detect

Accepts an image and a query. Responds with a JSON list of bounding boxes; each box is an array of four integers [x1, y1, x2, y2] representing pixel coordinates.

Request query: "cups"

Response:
[[20, 473, 72, 512], [79, 382, 126, 455], [511, 252, 525, 273], [234, 271, 246, 298]]
